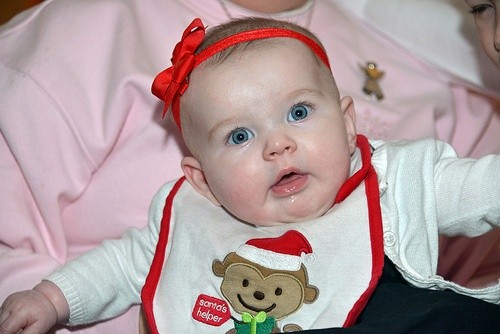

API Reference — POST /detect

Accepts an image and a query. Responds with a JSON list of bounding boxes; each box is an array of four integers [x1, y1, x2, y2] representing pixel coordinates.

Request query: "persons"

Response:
[[464, 0, 499, 68], [0, 0, 500, 334], [0, 16, 499, 334]]
